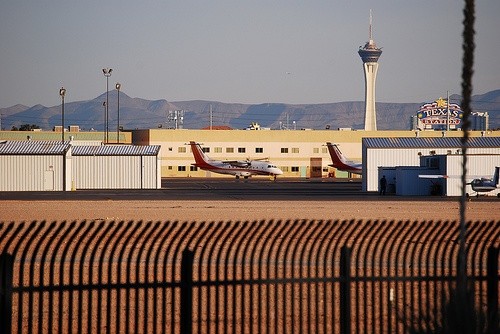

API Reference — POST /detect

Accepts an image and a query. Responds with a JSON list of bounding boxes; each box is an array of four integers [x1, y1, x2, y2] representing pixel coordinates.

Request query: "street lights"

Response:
[[59, 86, 67, 143], [103, 101, 107, 145], [102, 68, 113, 145], [115, 82, 122, 144]]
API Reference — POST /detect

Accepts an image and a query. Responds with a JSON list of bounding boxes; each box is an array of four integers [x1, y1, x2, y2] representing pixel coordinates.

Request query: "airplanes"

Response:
[[321, 142, 363, 182], [184, 141, 283, 179], [465, 166, 500, 199]]
